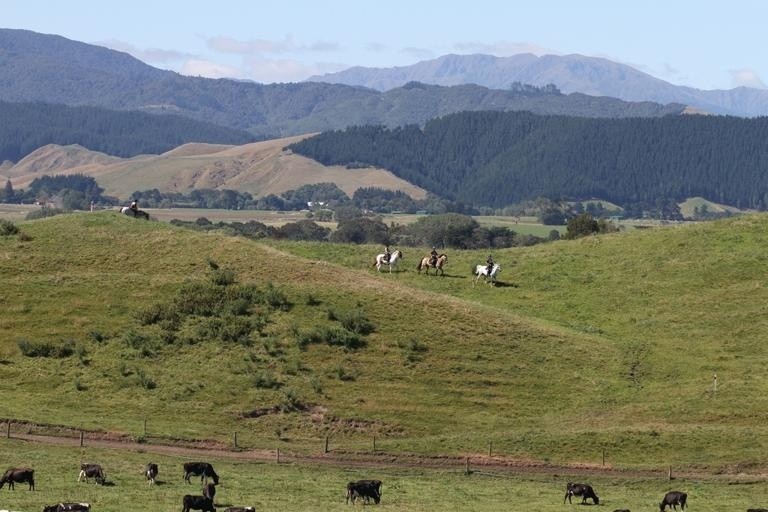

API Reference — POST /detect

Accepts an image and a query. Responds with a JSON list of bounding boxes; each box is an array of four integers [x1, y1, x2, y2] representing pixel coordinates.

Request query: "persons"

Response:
[[486, 253, 493, 274], [430, 246, 438, 265], [384, 244, 391, 263], [130, 199, 138, 217]]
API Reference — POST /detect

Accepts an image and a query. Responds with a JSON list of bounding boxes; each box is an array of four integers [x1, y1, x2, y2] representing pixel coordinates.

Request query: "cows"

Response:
[[78, 463, 106, 486], [203, 483, 216, 503], [145, 462, 158, 489], [182, 461, 219, 485], [0, 468, 35, 491], [346, 479, 383, 505], [43, 502, 92, 512], [224, 506, 255, 512], [659, 491, 688, 512], [182, 494, 217, 512], [564, 482, 599, 505]]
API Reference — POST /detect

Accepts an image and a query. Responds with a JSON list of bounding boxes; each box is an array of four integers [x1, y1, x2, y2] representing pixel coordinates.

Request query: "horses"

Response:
[[118, 207, 150, 221], [474, 263, 503, 289], [373, 250, 403, 274], [416, 254, 449, 276]]
